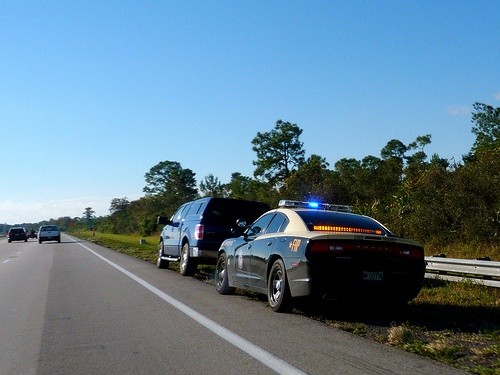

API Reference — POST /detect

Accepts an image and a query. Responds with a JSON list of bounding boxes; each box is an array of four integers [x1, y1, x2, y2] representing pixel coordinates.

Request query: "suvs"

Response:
[[37, 225, 62, 244], [155, 197, 271, 276], [5, 226, 27, 243]]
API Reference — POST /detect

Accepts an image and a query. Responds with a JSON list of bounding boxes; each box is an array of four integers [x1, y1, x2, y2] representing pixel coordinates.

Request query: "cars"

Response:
[[213, 200, 427, 312]]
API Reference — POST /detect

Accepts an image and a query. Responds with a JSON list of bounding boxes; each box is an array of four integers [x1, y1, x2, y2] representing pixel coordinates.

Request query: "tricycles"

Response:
[[26, 230, 37, 240]]
